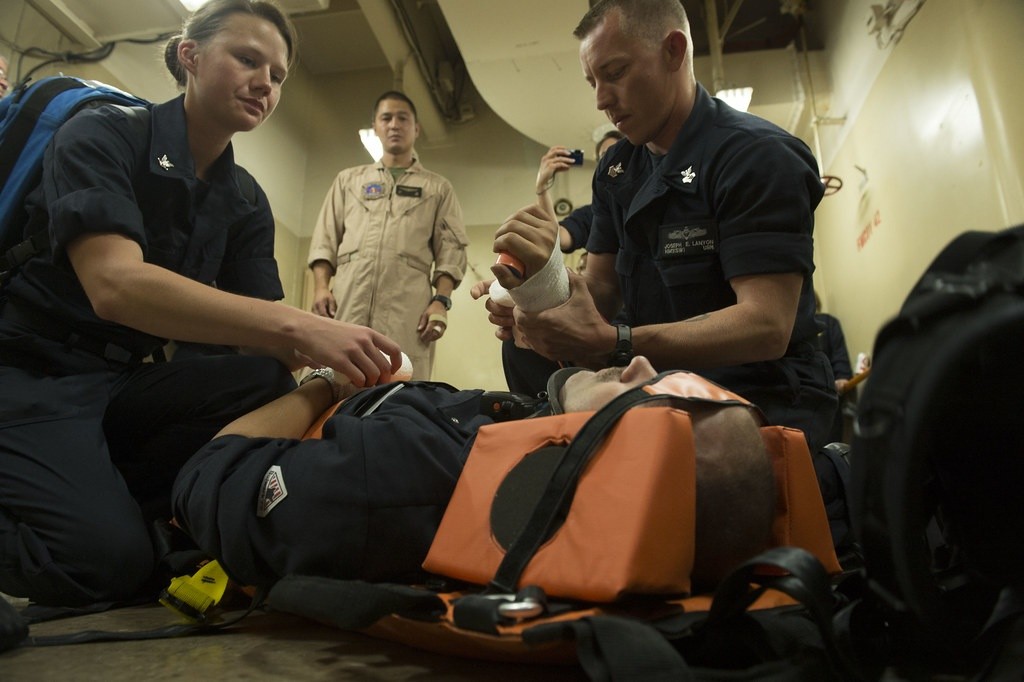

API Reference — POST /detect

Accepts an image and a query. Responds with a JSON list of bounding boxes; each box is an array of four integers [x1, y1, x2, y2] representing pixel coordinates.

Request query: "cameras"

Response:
[[561, 150, 584, 165]]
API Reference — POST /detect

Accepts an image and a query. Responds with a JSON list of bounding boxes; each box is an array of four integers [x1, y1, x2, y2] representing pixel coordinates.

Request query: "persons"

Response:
[[811, 294, 858, 443], [470, 0, 861, 551], [172, 204, 778, 606], [535, 131, 627, 254], [307, 90, 469, 383], [0, 0, 402, 607]]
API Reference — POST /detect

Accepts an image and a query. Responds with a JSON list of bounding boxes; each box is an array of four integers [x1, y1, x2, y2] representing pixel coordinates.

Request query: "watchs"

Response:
[[608, 324, 634, 366], [299, 369, 341, 404]]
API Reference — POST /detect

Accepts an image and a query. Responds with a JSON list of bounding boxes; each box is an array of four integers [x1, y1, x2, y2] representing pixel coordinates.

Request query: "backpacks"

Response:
[[848, 226, 1024, 659], [0, 75, 151, 234]]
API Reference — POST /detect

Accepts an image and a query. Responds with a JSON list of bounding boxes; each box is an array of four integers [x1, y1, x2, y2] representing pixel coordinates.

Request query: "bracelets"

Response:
[[432, 294, 452, 310]]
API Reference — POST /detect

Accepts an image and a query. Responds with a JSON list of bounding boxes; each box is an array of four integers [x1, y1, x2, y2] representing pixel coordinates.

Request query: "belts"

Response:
[[1, 294, 144, 370], [784, 326, 828, 357]]
